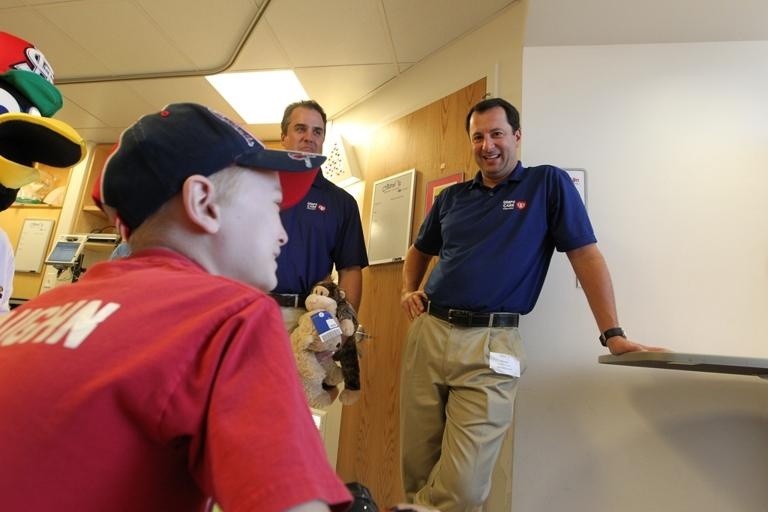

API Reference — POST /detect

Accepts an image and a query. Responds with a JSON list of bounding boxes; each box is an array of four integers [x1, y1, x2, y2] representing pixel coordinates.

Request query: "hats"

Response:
[[99, 105, 326, 239]]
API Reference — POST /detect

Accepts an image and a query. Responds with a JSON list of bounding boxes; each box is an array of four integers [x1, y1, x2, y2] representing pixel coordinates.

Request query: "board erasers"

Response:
[[30, 270, 35, 272], [392, 257, 401, 261]]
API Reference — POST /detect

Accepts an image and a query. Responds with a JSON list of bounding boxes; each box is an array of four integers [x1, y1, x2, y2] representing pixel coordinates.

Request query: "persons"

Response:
[[396, 95, 679, 511], [0, 102, 355, 511], [267, 100, 370, 428]]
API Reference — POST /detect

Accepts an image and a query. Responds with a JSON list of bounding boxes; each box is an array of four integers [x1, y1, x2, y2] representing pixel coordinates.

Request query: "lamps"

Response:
[[321, 136, 361, 188]]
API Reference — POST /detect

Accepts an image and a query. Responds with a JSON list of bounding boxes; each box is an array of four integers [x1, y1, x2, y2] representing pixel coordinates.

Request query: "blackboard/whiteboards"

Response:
[[14, 218, 55, 273]]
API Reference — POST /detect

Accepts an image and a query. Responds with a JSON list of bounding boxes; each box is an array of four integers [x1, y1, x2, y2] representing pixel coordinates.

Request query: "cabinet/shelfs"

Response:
[[71, 143, 122, 250], [0, 202, 63, 306]]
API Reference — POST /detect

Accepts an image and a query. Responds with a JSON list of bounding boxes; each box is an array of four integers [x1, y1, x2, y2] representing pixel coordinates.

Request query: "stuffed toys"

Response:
[[303, 279, 362, 405], [288, 309, 344, 411]]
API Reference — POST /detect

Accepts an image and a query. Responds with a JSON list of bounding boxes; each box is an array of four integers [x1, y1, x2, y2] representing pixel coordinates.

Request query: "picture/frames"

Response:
[[425, 173, 464, 220]]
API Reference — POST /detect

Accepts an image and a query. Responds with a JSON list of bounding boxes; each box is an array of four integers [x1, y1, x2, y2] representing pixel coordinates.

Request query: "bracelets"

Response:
[[597, 325, 629, 348]]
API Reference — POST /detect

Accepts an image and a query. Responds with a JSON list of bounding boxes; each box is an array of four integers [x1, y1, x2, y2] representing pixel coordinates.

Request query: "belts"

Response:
[[271, 292, 308, 308], [421, 299, 520, 328]]
